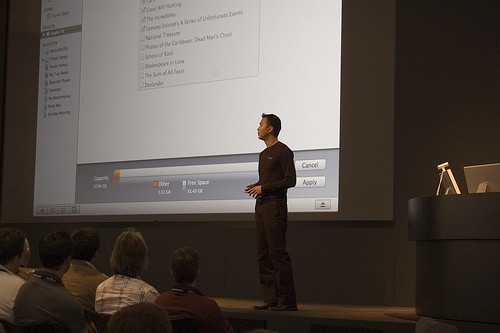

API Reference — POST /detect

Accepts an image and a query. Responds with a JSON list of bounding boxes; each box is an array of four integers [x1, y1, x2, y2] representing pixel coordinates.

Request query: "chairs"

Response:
[[84, 307, 114, 333], [171, 315, 206, 333]]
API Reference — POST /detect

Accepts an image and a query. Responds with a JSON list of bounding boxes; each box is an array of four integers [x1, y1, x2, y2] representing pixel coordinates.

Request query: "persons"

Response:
[[13, 230, 104, 333], [107, 302, 173, 333], [15, 238, 34, 280], [95, 227, 161, 316], [154, 247, 235, 333], [244, 113, 298, 311], [62, 226, 110, 310], [0, 227, 27, 333]]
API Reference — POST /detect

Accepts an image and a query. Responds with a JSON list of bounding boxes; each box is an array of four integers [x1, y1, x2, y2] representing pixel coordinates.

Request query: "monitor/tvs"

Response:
[[463, 163, 500, 193]]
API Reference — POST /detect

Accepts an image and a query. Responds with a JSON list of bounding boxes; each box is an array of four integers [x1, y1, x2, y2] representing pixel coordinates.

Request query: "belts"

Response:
[[256, 195, 282, 202]]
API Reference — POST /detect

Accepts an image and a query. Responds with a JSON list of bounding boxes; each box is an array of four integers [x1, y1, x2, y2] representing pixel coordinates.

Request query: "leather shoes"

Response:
[[268, 305, 298, 312], [254, 302, 276, 310]]
